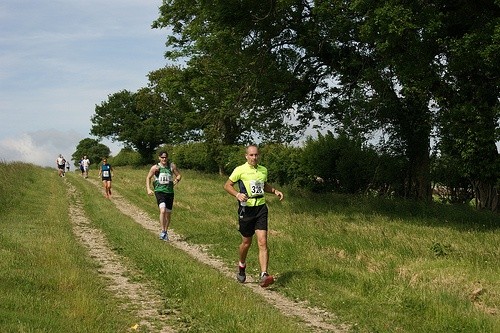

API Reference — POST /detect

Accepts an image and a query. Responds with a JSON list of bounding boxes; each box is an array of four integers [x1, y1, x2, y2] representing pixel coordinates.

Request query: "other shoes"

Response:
[[109, 190, 111, 194]]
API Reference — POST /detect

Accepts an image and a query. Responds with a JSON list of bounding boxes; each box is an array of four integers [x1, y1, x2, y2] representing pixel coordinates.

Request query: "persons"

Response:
[[224, 145, 283, 288], [57, 154, 90, 180], [146, 151, 181, 241], [99, 158, 113, 198]]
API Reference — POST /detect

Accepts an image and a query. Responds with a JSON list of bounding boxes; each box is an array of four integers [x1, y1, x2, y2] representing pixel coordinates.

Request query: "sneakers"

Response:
[[237, 264, 246, 283], [260, 272, 273, 287], [160, 231, 169, 241]]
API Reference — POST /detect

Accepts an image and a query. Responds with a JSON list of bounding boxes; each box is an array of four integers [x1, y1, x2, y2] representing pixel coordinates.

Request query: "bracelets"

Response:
[[175, 179, 179, 182], [236, 192, 240, 199]]
